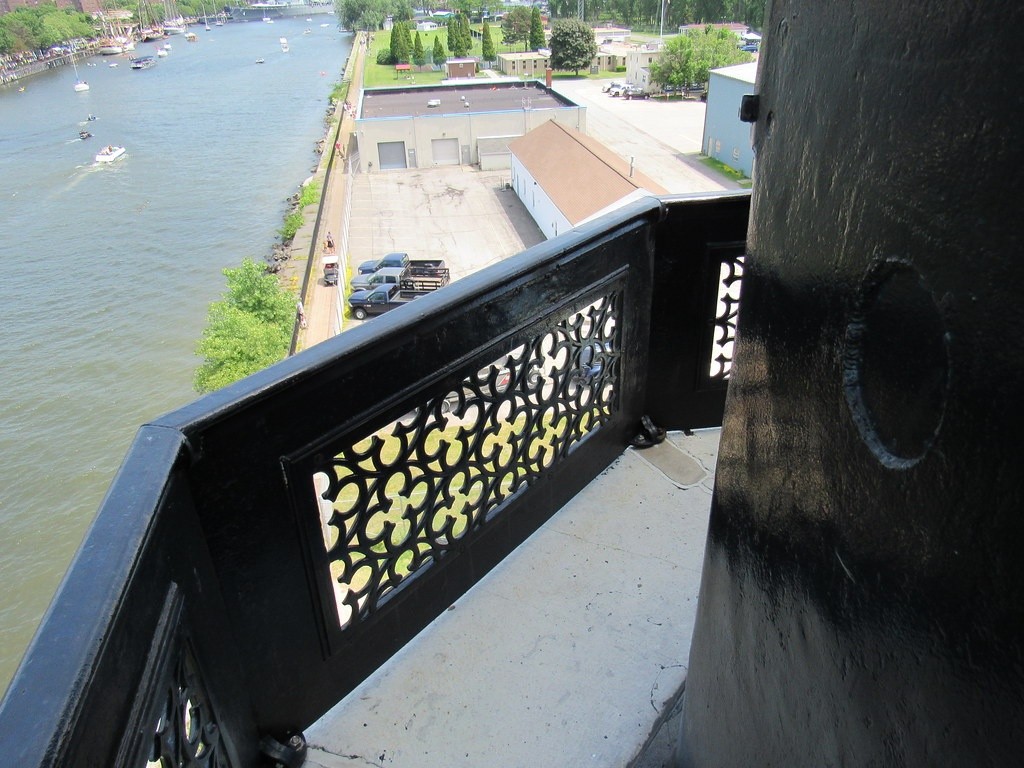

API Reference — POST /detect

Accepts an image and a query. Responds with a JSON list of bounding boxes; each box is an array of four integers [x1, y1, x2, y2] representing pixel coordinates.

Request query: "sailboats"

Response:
[[68, 50, 90, 92]]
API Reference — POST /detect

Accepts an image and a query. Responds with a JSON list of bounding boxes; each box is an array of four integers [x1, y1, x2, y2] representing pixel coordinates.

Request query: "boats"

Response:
[[18, 87, 24, 91], [96, 145, 127, 163], [67, 0, 355, 74]]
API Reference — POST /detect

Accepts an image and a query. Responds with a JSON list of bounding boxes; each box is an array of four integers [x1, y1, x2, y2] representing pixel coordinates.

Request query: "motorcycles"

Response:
[[79, 131, 92, 139], [87, 114, 96, 121]]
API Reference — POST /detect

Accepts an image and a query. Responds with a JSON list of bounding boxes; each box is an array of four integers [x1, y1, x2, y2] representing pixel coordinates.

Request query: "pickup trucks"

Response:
[[357, 253, 445, 276], [350, 267, 450, 292], [348, 285, 432, 320]]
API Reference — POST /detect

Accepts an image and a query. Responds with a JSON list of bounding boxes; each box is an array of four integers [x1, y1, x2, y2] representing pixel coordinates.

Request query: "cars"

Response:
[[739, 45, 758, 53], [412, 352, 546, 416], [610, 84, 635, 97], [624, 89, 650, 100], [570, 335, 612, 380], [602, 81, 620, 93]]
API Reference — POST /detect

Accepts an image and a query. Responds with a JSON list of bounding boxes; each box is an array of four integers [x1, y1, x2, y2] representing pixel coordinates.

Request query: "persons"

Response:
[[327, 231, 334, 253], [335, 140, 341, 153], [79, 112, 118, 155], [324, 239, 327, 252]]
